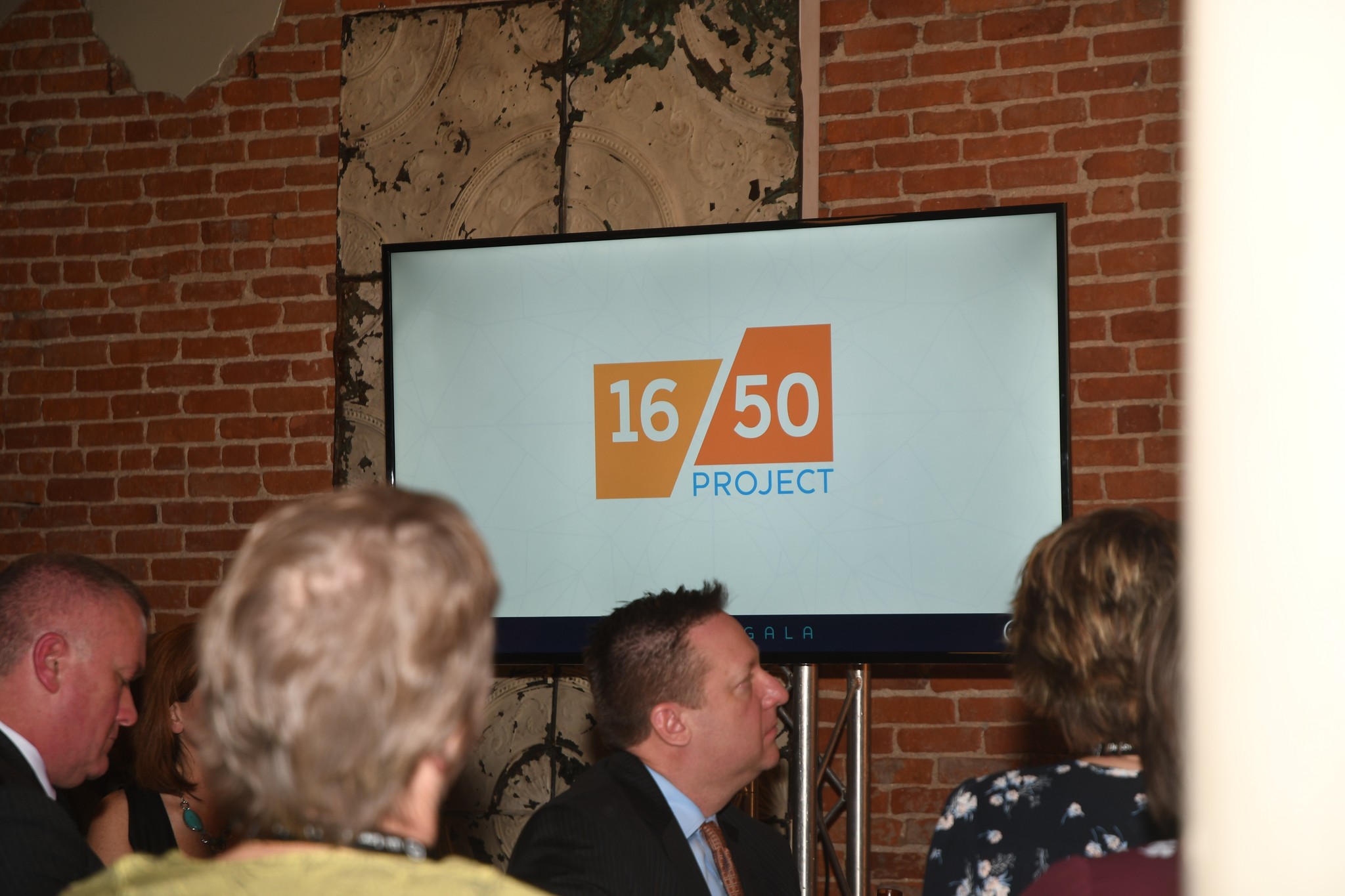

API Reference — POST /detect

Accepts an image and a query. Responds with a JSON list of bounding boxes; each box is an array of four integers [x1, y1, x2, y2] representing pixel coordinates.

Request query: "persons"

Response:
[[921, 507, 1176, 896], [1018, 571, 1187, 896], [85, 621, 240, 869], [59, 484, 552, 896], [505, 580, 802, 896], [0, 551, 149, 896]]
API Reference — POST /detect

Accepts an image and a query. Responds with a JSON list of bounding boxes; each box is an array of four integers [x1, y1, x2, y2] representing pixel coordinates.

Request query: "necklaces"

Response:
[[180, 796, 234, 855]]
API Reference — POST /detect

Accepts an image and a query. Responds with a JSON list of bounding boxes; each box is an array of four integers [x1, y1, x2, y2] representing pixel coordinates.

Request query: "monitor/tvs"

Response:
[[381, 201, 1068, 660]]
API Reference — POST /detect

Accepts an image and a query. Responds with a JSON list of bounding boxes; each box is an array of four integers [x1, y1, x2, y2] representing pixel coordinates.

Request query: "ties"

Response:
[[699, 821, 746, 896]]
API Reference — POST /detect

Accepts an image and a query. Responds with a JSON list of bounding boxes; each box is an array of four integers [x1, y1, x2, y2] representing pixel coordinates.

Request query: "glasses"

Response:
[[1002, 618, 1016, 646]]
